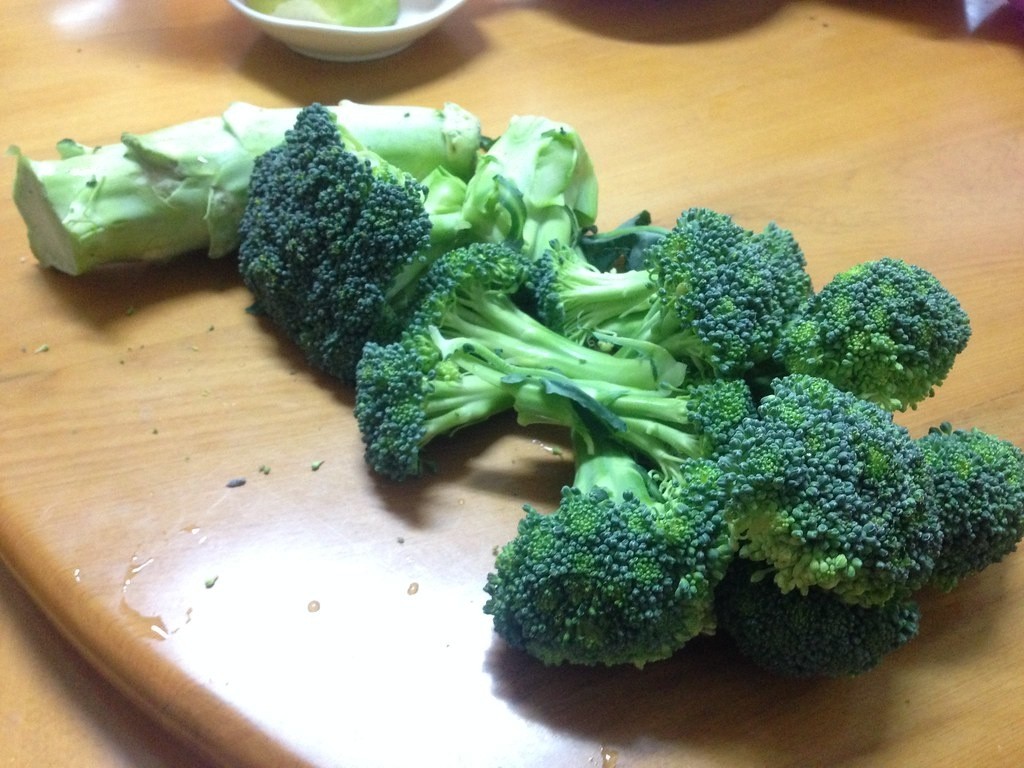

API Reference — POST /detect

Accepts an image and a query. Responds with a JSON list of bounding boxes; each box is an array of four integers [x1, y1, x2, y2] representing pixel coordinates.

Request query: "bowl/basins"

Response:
[[227, 0, 470, 62]]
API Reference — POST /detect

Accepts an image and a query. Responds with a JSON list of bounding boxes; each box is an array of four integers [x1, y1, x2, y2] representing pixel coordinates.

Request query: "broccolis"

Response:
[[11, 100, 1024, 675]]
